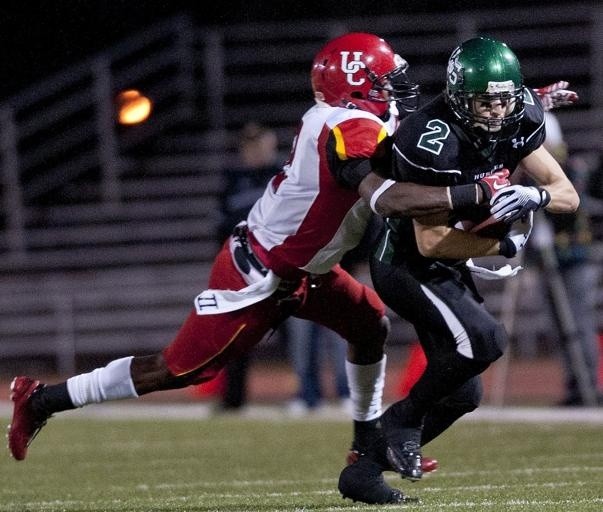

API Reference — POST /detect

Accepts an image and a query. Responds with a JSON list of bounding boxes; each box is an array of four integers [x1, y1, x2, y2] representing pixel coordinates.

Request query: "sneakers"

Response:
[[338, 399, 438, 505], [7, 375, 47, 461]]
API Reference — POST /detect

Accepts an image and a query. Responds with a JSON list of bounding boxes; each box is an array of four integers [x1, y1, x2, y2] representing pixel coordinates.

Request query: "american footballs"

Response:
[[456, 201, 512, 240]]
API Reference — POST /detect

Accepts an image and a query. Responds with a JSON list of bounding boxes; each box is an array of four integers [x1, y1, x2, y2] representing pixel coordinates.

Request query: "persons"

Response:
[[403, 37, 580, 504], [10, 33, 578, 461], [212, 126, 352, 417]]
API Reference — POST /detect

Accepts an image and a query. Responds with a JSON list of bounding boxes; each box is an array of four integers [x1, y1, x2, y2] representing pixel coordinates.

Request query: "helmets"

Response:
[[446, 36, 522, 100], [311, 32, 411, 117]]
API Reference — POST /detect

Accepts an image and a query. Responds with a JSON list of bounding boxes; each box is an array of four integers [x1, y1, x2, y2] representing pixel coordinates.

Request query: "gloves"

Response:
[[475, 168, 552, 259], [532, 81, 578, 111]]
